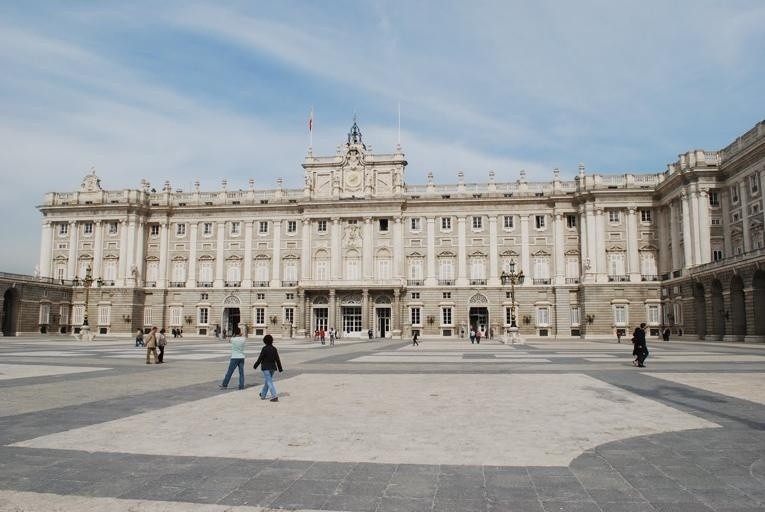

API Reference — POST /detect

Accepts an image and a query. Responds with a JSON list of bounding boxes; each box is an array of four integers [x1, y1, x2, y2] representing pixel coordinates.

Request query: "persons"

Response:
[[636, 323, 649, 367], [218, 327, 245, 390], [329, 328, 335, 347], [368, 329, 373, 339], [661, 326, 671, 341], [253, 334, 283, 401], [314, 328, 326, 345], [632, 328, 640, 366], [412, 331, 419, 346], [475, 331, 481, 345], [136, 326, 184, 365], [470, 328, 475, 344], [214, 322, 239, 343]]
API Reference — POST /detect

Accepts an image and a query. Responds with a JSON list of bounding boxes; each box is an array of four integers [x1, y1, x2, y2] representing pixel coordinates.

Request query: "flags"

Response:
[[308, 111, 313, 131]]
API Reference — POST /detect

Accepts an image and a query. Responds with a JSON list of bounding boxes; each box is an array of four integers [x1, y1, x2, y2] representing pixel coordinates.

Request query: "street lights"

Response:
[[73, 266, 102, 327], [500, 260, 524, 328]]
